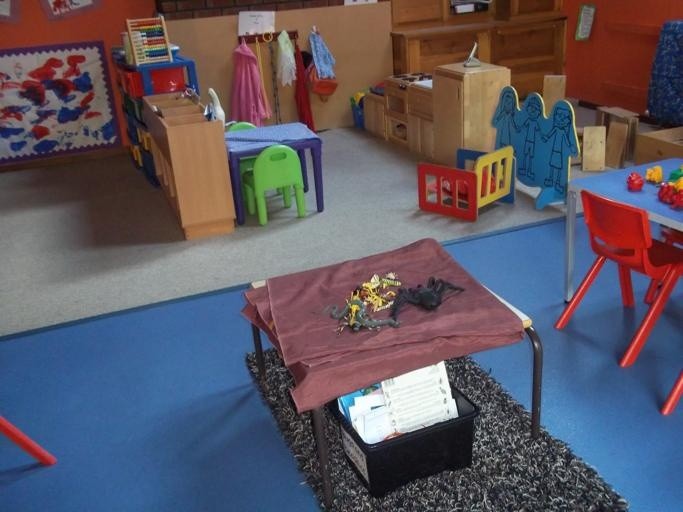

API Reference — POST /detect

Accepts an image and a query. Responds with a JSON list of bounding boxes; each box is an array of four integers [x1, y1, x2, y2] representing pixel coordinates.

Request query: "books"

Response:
[[338, 361, 459, 439]]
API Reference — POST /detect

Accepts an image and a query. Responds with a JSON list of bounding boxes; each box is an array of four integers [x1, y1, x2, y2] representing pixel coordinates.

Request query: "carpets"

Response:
[[246, 348, 629, 512], [1, 214, 683, 512]]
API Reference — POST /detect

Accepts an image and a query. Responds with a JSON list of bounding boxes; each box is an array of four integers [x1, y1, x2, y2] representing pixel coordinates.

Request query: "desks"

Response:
[[249, 238, 543, 509], [225, 122, 323, 224], [565, 157, 683, 301]]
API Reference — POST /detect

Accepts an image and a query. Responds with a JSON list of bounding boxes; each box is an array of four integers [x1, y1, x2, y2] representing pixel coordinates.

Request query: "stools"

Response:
[[660, 371, 682, 415], [644, 228, 683, 303]]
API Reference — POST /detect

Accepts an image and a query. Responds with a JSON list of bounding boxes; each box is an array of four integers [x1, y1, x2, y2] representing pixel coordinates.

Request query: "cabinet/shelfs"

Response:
[[360, 59, 510, 165], [112, 45, 236, 241], [393, 0, 567, 110]]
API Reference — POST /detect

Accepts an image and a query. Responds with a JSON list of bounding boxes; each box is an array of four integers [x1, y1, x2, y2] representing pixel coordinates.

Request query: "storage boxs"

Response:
[[634, 126, 683, 164], [326, 378, 480, 497]]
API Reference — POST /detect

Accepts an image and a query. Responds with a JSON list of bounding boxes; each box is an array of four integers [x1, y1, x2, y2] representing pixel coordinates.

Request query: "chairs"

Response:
[[555, 191, 683, 368], [242, 144, 304, 225], [230, 122, 260, 173]]
